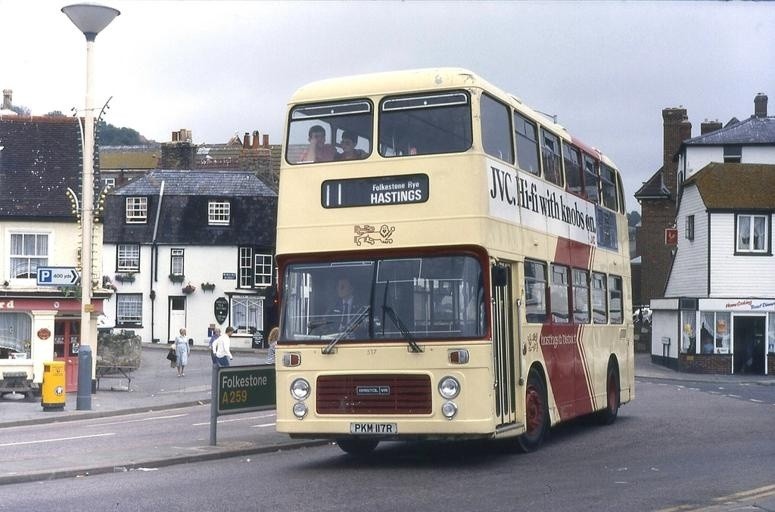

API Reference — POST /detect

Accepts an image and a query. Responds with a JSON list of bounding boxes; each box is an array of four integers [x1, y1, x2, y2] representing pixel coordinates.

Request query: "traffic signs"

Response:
[[36, 264, 80, 288], [219, 365, 276, 415]]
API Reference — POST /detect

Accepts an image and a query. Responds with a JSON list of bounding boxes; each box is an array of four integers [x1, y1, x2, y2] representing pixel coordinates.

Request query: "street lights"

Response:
[[58, 1, 120, 409]]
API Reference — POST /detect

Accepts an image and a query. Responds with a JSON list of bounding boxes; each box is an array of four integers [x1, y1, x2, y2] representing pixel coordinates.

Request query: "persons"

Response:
[[701, 337, 714, 354], [339, 129, 368, 160], [174, 327, 190, 377], [307, 275, 381, 338], [212, 327, 235, 368], [209, 327, 221, 364], [267, 326, 279, 365], [300, 124, 337, 161]]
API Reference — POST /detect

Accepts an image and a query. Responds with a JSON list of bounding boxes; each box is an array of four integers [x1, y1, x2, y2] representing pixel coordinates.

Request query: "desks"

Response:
[[1, 370, 39, 403], [97, 364, 136, 393]]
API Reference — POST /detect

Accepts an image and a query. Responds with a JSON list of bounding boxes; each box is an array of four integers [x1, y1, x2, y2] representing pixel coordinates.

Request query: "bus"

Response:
[[274, 67, 637, 455]]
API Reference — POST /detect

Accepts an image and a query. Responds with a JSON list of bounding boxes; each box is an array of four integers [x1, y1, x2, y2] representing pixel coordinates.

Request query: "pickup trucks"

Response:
[[0, 358, 38, 406]]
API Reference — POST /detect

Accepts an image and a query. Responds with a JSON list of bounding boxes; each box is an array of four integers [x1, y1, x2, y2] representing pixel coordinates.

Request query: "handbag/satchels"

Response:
[[167, 350, 176, 362]]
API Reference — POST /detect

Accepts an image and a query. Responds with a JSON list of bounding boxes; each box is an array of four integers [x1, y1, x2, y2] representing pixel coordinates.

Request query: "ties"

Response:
[[343, 303, 347, 328]]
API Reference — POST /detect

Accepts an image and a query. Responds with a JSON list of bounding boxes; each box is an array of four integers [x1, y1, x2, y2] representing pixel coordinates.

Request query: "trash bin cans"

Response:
[[41, 361, 66, 412]]
